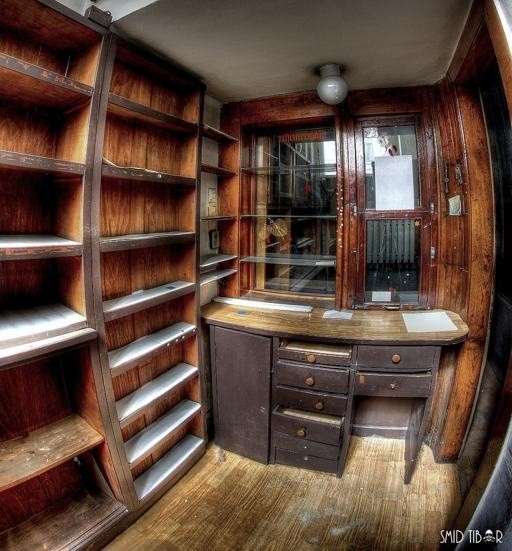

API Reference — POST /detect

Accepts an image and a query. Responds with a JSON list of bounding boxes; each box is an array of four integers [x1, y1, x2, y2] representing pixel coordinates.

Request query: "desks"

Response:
[[200, 300, 470, 484]]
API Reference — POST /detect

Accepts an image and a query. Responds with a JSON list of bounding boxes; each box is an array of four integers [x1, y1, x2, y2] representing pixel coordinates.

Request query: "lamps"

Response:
[[318, 63, 348, 104]]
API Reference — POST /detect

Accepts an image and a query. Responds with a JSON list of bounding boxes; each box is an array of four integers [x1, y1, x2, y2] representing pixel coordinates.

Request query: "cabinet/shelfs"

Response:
[[0, 0, 134, 550], [92, 30, 206, 512], [254, 136, 293, 292], [293, 141, 320, 293], [199, 123, 240, 285]]
